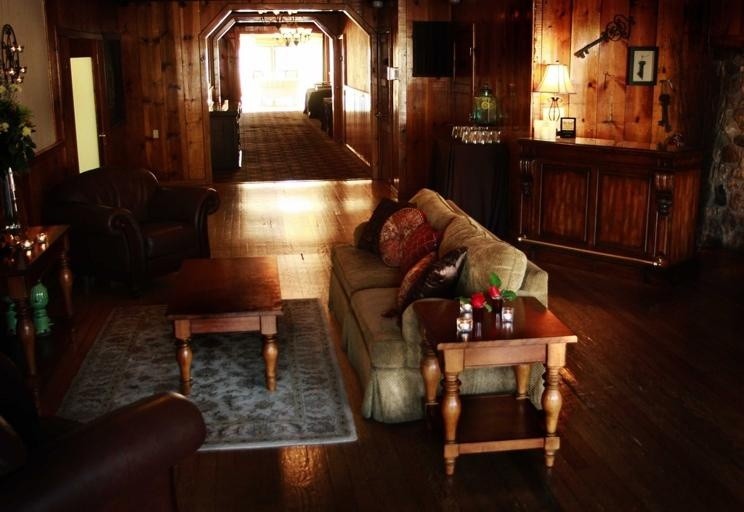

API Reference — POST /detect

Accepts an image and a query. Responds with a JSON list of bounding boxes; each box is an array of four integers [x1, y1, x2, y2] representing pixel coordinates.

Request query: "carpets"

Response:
[[50, 291, 362, 449], [219, 109, 373, 182]]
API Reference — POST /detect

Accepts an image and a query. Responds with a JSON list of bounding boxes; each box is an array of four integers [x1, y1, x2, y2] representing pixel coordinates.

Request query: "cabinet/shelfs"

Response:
[[510, 135, 704, 271], [433, 133, 508, 234], [212, 98, 245, 170]]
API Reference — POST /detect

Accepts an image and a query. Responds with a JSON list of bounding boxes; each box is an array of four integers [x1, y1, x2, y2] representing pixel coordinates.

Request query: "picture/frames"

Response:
[[626, 44, 658, 86]]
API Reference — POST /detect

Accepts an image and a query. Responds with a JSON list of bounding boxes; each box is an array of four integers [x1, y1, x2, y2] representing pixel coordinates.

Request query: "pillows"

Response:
[[394, 222, 439, 283], [379, 205, 424, 266], [355, 200, 418, 250], [393, 251, 434, 305], [398, 243, 470, 323]]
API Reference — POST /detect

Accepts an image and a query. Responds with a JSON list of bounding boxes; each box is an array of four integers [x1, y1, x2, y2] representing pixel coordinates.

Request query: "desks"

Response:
[[319, 97, 333, 136], [0, 219, 70, 414], [304, 87, 333, 118], [412, 290, 577, 479]]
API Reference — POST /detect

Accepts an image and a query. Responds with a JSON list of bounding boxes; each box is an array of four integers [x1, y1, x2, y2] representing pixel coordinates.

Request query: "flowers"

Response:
[[458, 291, 492, 312], [0, 81, 38, 178], [487, 270, 518, 303]]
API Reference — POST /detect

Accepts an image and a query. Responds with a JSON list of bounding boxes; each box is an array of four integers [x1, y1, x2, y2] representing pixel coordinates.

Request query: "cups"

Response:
[[501, 307, 514, 322], [458, 333, 470, 341], [501, 323, 514, 334], [456, 317, 473, 332], [451, 126, 500, 144]]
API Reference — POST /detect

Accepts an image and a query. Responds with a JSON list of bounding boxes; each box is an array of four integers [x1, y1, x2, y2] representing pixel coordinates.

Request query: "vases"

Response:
[[453, 303, 473, 333], [499, 304, 513, 322], [0, 164, 23, 232]]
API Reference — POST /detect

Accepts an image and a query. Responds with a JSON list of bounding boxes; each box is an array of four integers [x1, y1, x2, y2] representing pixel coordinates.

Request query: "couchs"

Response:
[[323, 186, 548, 425], [0, 374, 208, 511], [53, 164, 221, 298]]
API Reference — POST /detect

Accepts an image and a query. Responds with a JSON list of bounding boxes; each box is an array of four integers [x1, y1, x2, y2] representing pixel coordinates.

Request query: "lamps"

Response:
[[257, 8, 299, 31], [0, 23, 28, 92], [272, 23, 312, 46], [537, 59, 575, 140]]
